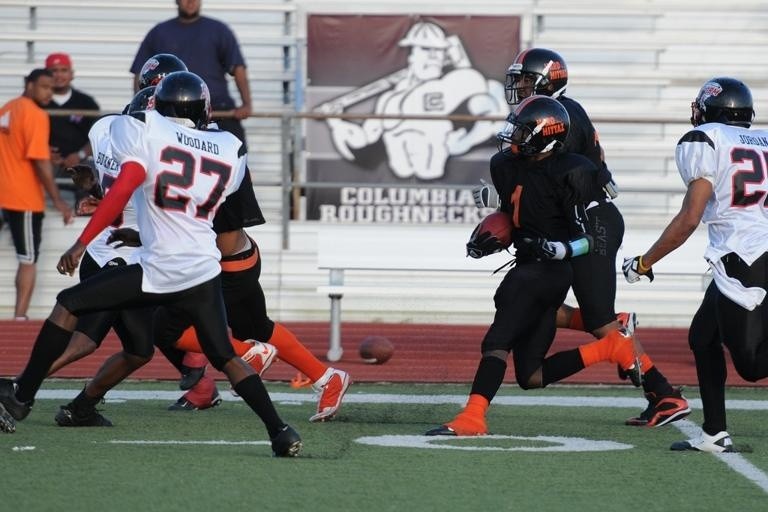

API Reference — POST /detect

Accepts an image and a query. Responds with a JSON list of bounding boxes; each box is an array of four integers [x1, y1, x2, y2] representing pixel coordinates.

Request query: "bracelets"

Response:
[[639, 254, 651, 271]]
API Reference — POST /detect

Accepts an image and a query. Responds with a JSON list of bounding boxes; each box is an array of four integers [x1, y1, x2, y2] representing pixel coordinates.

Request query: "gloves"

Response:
[[466, 223, 503, 258], [622, 257, 654, 283], [473, 179, 501, 208], [524, 236, 566, 262]]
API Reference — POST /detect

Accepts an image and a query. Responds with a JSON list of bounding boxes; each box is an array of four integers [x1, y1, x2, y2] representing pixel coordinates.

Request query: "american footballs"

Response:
[[359, 335, 394, 364], [478, 213, 513, 249]]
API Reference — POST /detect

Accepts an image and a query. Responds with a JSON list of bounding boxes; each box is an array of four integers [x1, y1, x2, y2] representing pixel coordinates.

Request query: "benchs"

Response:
[[316, 224, 713, 362]]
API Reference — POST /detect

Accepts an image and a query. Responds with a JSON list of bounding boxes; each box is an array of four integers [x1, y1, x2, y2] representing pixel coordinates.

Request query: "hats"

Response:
[[46, 54, 69, 68]]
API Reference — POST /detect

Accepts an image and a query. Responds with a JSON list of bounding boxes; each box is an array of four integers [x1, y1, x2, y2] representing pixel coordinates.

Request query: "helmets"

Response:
[[127, 54, 212, 127], [690, 79, 753, 127], [496, 95, 571, 159], [503, 47, 568, 105]]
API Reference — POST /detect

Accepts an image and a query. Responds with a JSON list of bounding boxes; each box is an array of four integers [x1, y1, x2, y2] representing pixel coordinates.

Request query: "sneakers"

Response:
[[0, 379, 35, 434], [624, 386, 691, 427], [609, 311, 645, 387], [168, 351, 223, 411], [267, 423, 303, 457], [55, 395, 113, 426], [229, 338, 278, 398], [425, 424, 487, 435], [308, 366, 353, 422], [670, 428, 733, 453]]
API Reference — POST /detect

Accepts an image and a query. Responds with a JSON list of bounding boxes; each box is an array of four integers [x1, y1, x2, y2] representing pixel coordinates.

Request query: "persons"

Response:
[[428, 94, 643, 436], [0, 52, 355, 458], [312, 20, 512, 182], [622, 78, 767, 453], [129, 1, 253, 155], [501, 47, 690, 427]]
